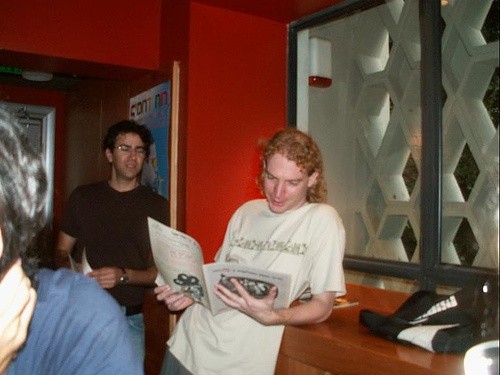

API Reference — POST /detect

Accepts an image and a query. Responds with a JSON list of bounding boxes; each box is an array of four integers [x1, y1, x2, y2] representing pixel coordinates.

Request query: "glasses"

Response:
[[114, 145, 146, 156]]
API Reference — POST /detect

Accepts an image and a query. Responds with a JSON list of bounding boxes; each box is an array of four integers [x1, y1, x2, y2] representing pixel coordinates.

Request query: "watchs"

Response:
[[118, 265, 128, 285]]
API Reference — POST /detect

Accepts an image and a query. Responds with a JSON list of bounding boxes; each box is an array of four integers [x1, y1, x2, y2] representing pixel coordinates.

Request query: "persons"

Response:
[[153, 129, 347, 375], [0, 107, 135, 375], [56, 119, 170, 316]]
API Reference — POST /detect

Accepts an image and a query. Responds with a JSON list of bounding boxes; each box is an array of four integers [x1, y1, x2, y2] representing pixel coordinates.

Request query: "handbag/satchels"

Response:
[[358, 286, 484, 354]]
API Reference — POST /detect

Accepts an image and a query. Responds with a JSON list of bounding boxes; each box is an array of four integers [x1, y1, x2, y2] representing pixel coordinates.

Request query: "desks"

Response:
[[275, 284, 467, 375]]
